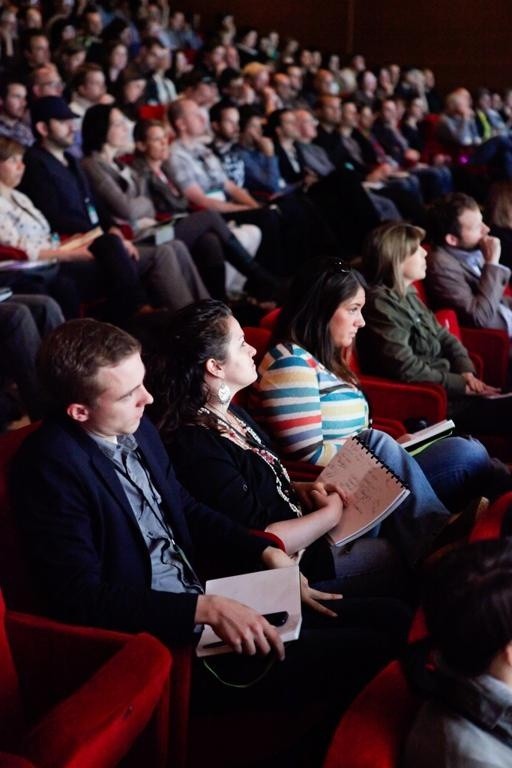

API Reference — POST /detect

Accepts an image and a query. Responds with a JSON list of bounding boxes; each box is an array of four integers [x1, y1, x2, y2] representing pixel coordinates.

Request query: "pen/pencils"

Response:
[[203, 640, 228, 649]]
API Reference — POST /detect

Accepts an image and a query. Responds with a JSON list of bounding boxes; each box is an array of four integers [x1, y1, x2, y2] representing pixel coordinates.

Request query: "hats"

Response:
[[31, 96, 79, 120]]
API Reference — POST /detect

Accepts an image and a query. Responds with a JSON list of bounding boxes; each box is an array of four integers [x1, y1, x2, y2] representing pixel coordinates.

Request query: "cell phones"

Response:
[[261, 611, 288, 627]]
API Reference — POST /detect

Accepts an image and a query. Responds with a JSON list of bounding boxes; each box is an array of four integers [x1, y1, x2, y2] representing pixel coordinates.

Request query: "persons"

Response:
[[2, 224, 511, 768], [0, 0, 512, 430]]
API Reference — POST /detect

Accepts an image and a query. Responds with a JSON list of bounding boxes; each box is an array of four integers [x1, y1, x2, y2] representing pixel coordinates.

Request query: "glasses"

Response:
[[323, 258, 352, 280]]
